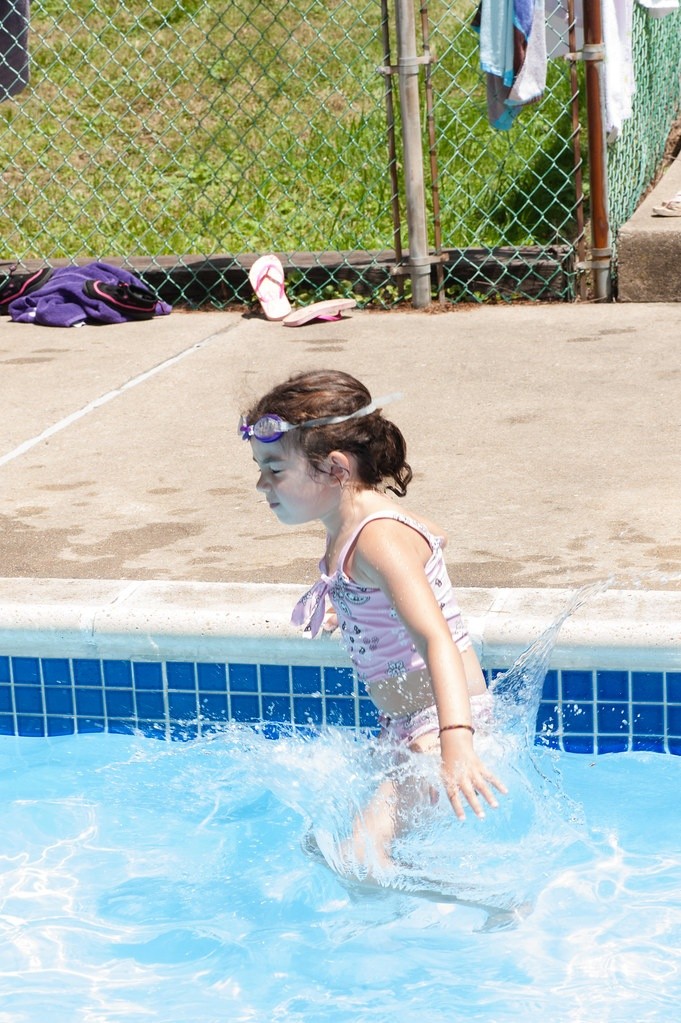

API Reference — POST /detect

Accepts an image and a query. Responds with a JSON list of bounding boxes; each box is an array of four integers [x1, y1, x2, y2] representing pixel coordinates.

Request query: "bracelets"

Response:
[[438, 724, 477, 736]]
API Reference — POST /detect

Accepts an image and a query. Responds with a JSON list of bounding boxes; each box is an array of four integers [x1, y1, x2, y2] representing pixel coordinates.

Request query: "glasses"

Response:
[[240, 402, 379, 443]]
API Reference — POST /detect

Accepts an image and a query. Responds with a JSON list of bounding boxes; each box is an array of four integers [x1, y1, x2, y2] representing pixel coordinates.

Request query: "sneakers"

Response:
[[0, 268, 53, 309], [84, 278, 156, 319]]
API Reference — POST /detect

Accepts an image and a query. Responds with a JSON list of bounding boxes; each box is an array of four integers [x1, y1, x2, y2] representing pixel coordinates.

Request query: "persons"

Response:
[[244, 366, 512, 880]]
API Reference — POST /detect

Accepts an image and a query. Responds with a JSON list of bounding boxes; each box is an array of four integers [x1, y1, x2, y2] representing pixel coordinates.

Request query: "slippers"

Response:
[[283, 298, 356, 326], [249, 255, 293, 320]]
